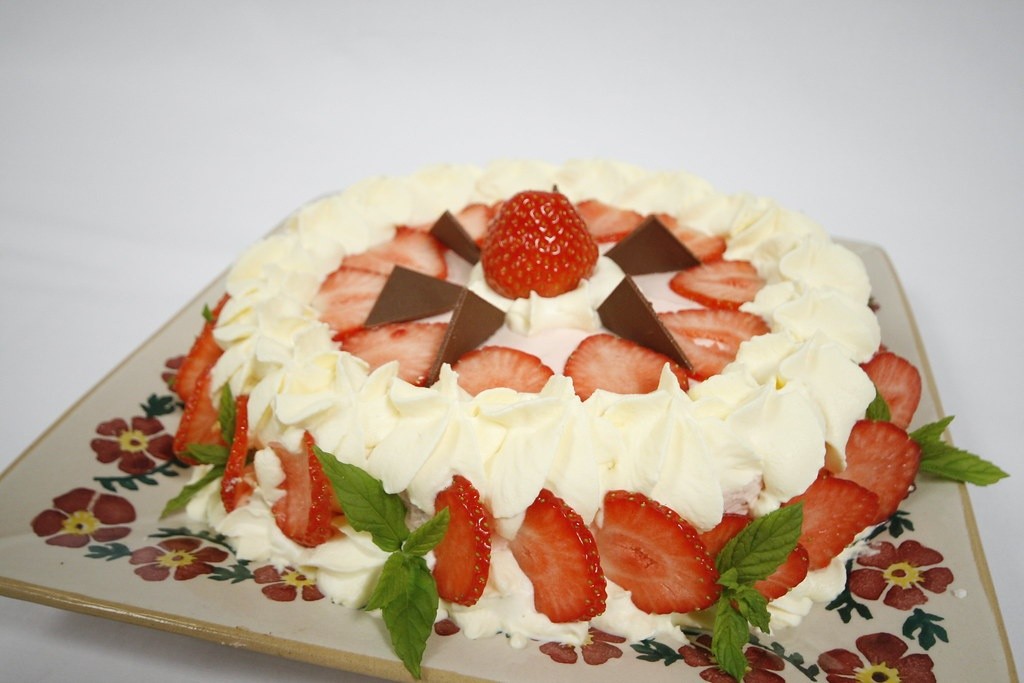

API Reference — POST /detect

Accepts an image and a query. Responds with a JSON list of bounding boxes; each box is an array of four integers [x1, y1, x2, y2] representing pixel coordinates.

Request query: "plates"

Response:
[[0, 191, 1024, 683]]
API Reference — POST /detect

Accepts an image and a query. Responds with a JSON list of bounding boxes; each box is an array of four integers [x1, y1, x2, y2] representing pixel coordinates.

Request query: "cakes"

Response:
[[173, 157, 1012, 683]]
